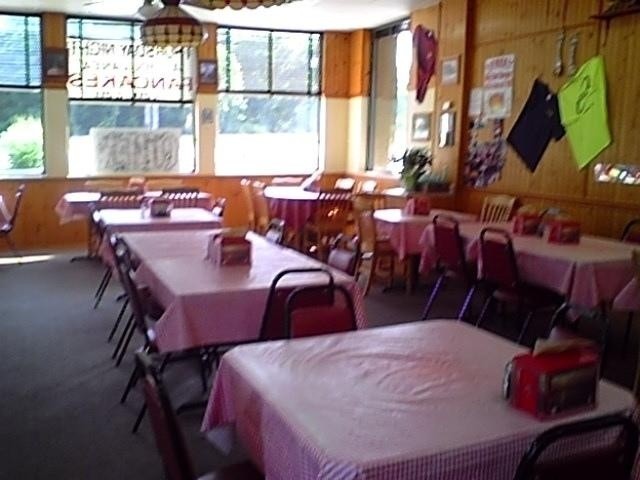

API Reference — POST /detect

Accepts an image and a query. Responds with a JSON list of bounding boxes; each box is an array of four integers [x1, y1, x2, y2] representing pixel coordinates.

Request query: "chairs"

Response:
[[1, 183, 26, 270]]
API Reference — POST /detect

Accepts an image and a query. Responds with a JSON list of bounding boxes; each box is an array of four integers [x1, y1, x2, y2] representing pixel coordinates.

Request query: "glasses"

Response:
[[138, 0, 204, 50]]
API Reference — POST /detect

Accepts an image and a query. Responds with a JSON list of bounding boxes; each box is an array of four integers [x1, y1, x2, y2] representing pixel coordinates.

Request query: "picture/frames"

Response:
[[410, 111, 431, 142], [439, 54, 460, 88]]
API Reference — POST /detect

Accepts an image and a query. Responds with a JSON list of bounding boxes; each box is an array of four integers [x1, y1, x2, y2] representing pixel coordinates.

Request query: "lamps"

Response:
[[1, 183, 26, 270], [138, 0, 204, 50]]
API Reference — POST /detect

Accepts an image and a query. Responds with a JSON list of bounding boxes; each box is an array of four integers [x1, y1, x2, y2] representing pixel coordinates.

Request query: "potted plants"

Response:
[[439, 54, 460, 88], [410, 111, 431, 142]]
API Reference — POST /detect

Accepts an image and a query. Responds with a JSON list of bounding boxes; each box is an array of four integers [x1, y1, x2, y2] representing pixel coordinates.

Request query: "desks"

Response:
[[54, 173, 639, 479]]
